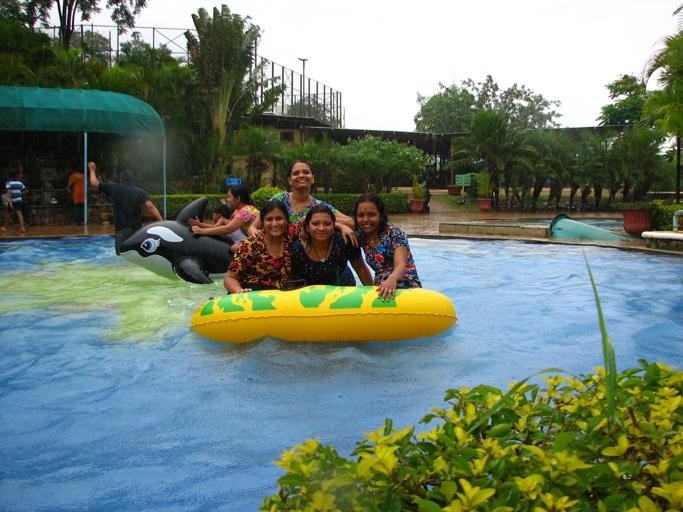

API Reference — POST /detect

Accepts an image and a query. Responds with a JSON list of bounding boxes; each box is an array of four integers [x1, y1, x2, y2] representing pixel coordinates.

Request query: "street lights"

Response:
[[297, 56, 308, 118]]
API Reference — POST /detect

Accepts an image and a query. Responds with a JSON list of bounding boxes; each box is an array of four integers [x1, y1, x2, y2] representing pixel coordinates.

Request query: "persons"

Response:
[[186, 202, 246, 244], [87, 161, 163, 256], [296, 193, 421, 303], [0, 169, 28, 233], [189, 183, 260, 238], [287, 205, 374, 287], [64, 164, 83, 225], [245, 157, 356, 241], [223, 200, 360, 297]]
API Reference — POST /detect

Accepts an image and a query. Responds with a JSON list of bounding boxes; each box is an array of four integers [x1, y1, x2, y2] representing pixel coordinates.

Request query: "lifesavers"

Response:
[[190, 286, 458, 343]]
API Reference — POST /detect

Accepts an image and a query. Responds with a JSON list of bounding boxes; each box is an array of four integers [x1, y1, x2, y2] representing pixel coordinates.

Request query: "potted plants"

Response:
[[477, 170, 494, 211], [621, 201, 656, 236], [409, 174, 428, 213]]
